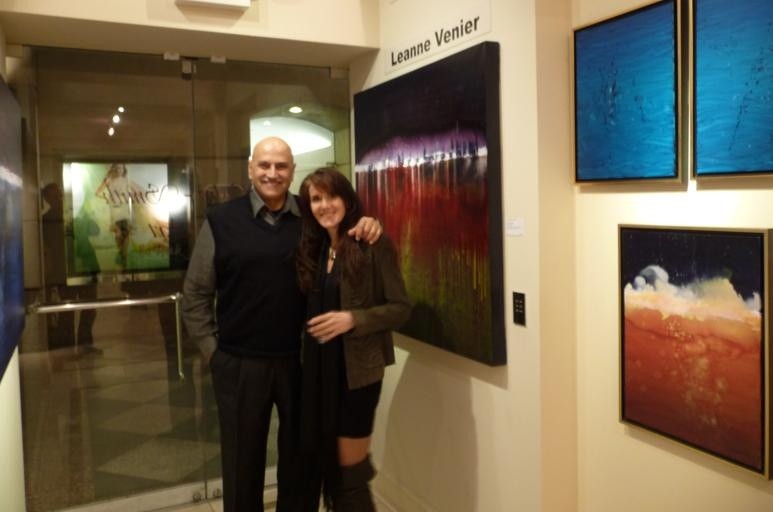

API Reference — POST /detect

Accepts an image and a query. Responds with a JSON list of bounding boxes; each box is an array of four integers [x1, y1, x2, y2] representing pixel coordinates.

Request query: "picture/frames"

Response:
[[618, 224, 773, 481], [570, 0, 683, 185], [688, 0, 773, 180]]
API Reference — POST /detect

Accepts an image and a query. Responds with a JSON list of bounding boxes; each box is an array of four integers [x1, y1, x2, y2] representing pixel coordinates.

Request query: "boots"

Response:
[[320, 451, 377, 512]]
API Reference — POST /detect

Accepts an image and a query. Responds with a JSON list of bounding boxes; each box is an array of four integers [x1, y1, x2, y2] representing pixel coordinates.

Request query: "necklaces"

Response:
[[327, 240, 337, 261]]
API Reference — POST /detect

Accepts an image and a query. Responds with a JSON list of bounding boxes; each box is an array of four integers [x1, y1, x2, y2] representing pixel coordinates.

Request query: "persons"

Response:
[[176, 135, 384, 511], [295, 166, 414, 511], [97, 162, 143, 268], [42, 184, 100, 359], [140, 166, 214, 440]]
